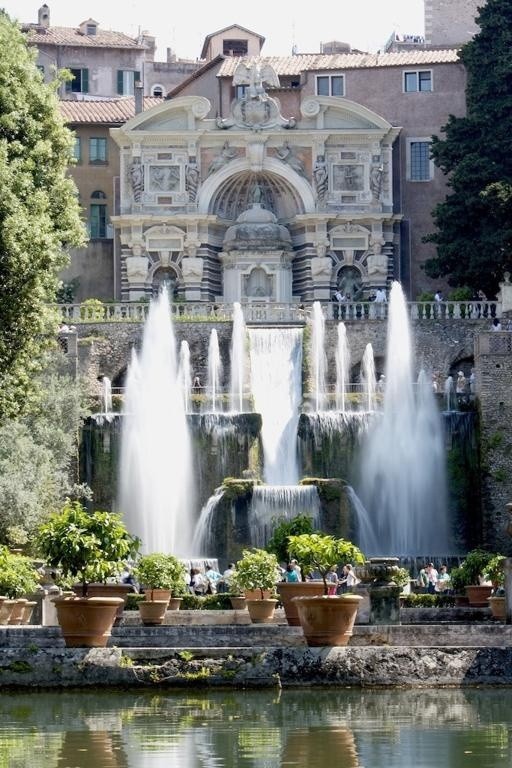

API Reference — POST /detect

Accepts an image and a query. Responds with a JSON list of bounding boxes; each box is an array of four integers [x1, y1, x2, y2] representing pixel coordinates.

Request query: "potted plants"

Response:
[[0, 543, 44, 625], [463, 549, 494, 604], [227, 547, 281, 623], [483, 554, 509, 616], [267, 515, 336, 626], [138, 550, 189, 626], [31, 500, 141, 648], [288, 531, 366, 647]]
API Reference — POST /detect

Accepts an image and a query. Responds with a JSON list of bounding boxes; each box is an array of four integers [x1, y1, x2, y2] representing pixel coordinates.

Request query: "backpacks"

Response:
[[196, 579, 209, 592]]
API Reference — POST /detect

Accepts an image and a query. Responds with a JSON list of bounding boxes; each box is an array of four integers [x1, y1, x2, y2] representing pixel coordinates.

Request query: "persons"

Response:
[[367, 290, 376, 301], [185, 155, 200, 191], [327, 564, 357, 595], [124, 566, 136, 586], [339, 269, 362, 299], [434, 290, 444, 313], [124, 238, 150, 282], [417, 563, 450, 594], [129, 157, 142, 188], [434, 366, 475, 393], [342, 289, 352, 303], [159, 272, 176, 296], [183, 563, 236, 596], [478, 287, 488, 313], [334, 286, 346, 318], [313, 155, 328, 187], [370, 154, 383, 186], [378, 373, 387, 390], [372, 286, 387, 302], [192, 375, 204, 393], [368, 236, 391, 281], [272, 140, 305, 171], [277, 559, 302, 584], [479, 574, 504, 592], [208, 139, 239, 173], [183, 239, 204, 279], [310, 236, 333, 279]]
